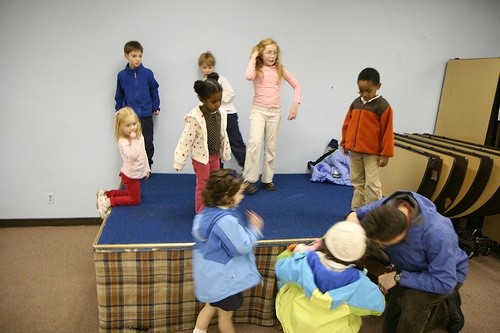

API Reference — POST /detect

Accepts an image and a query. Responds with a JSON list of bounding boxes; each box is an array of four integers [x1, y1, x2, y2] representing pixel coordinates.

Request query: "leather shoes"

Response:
[[246, 183, 257, 194], [261, 182, 276, 191]]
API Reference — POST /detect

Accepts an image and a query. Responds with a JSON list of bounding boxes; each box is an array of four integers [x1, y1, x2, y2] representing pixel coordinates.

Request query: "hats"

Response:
[[324, 221, 367, 262]]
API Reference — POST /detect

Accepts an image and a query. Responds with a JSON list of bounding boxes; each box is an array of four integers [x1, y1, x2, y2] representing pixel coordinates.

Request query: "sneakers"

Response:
[[98, 196, 109, 219], [96, 190, 105, 209]]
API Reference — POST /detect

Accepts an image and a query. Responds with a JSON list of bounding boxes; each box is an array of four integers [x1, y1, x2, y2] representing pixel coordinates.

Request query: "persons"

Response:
[[191, 168, 264, 333], [174, 72, 232, 215], [340, 68, 394, 219], [242, 38, 301, 193], [96, 107, 152, 220], [344, 190, 469, 333], [198, 51, 246, 171], [115, 41, 160, 179], [273, 221, 386, 333]]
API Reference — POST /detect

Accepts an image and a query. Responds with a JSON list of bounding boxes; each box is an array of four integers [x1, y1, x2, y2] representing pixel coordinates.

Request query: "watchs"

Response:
[[394, 270, 401, 286]]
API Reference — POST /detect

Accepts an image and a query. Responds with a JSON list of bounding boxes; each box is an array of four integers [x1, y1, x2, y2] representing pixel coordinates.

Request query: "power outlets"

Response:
[[48, 193, 55, 204]]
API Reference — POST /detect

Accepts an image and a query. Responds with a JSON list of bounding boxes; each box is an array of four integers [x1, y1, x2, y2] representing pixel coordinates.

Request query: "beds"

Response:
[[93, 171, 383, 333]]
